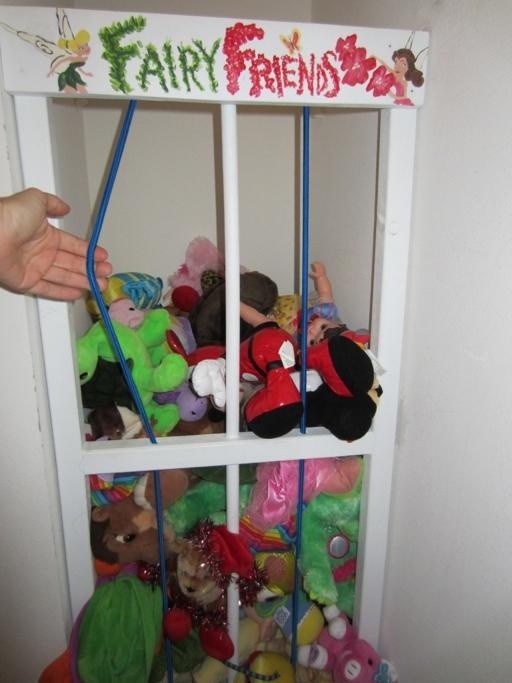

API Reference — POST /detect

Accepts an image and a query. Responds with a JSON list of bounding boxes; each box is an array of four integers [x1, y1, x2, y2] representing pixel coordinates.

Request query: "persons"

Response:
[[0, 189, 111, 303]]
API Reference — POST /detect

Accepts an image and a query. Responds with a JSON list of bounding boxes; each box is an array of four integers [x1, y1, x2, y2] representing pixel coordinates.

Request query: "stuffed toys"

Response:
[[74, 236, 385, 442], [39, 453, 398, 682], [73, 235, 385, 443], [38, 453, 398, 682]]
